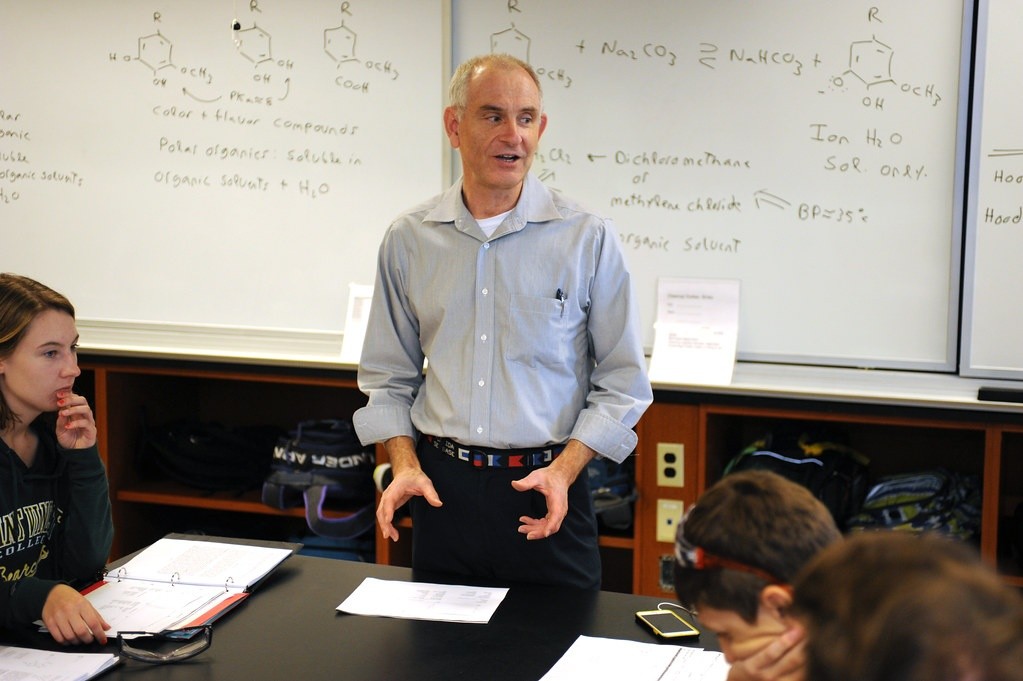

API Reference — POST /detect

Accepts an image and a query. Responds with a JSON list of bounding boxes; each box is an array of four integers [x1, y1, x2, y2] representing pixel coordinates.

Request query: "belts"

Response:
[[426, 435, 566, 468]]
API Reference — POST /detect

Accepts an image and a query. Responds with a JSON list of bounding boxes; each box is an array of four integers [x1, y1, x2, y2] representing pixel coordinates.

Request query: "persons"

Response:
[[793, 530, 1023, 681], [352, 54, 654, 591], [663, 469, 842, 681], [0, 274, 114, 643]]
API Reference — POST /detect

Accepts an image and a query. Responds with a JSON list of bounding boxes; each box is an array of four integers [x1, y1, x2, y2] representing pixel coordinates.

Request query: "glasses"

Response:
[[674, 504, 794, 600], [117, 625, 213, 664]]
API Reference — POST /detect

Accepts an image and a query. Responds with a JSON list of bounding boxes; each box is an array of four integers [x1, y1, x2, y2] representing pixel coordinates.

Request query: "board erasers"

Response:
[[977, 386, 1023, 403]]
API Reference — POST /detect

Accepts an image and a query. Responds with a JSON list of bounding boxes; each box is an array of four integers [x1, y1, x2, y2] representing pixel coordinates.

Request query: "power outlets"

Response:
[[656, 442, 684, 488]]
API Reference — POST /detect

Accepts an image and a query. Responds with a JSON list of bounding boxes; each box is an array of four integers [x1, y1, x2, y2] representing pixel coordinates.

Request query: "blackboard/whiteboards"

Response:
[[957, 1, 1023, 380], [0, 1, 449, 343], [451, 0, 974, 378]]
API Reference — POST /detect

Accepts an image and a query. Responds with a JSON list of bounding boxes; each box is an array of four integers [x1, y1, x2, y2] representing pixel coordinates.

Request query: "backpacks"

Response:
[[846, 471, 982, 554], [262, 418, 376, 541]]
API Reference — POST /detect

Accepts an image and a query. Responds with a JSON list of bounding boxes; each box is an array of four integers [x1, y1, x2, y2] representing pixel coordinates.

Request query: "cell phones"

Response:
[[636, 609, 700, 642]]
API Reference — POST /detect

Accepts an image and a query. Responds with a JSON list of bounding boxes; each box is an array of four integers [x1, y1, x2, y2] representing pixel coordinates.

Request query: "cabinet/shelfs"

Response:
[[72, 361, 1023, 605]]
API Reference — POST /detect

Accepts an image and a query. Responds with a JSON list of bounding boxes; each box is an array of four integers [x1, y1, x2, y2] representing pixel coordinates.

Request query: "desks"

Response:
[[1, 555, 734, 681]]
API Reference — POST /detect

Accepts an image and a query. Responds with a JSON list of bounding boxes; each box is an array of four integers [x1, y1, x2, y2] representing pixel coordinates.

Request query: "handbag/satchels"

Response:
[[134, 416, 289, 491], [721, 434, 870, 532]]
